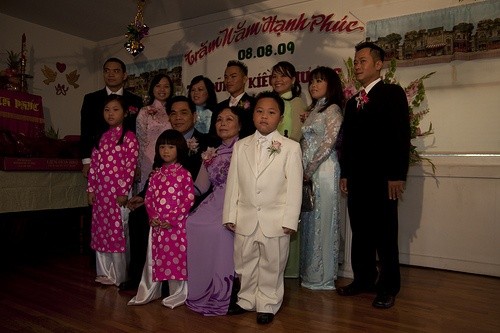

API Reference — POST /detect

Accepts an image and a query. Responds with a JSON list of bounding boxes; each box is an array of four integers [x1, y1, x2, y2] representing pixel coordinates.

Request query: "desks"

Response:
[[0, 169, 97, 289]]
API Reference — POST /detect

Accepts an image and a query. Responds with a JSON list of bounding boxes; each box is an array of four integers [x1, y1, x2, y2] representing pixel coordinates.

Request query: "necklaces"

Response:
[[160, 165, 182, 176]]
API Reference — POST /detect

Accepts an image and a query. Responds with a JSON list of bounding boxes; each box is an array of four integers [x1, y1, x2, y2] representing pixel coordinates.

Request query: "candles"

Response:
[[21, 33, 27, 59]]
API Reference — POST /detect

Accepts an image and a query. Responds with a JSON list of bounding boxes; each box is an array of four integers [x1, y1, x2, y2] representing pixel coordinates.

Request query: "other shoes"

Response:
[[229, 303, 246, 315], [256, 312, 275, 325], [372, 293, 396, 309], [336, 281, 376, 297], [94, 276, 116, 286]]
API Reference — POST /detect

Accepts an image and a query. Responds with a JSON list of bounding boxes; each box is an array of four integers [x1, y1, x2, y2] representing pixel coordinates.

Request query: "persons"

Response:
[[186, 74, 220, 133], [338, 42, 411, 309], [143, 129, 193, 305], [299, 67, 345, 293], [87, 94, 139, 285], [136, 74, 175, 191], [210, 60, 255, 137], [128, 96, 212, 283], [270, 62, 309, 142], [223, 90, 302, 324], [187, 105, 243, 315], [81, 58, 144, 268]]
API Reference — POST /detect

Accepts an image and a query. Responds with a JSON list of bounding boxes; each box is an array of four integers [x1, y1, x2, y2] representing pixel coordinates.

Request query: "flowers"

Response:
[[185, 136, 200, 156], [299, 56, 439, 202], [126, 105, 140, 117], [200, 146, 217, 173], [148, 108, 158, 121], [355, 89, 369, 110], [266, 140, 281, 158], [238, 100, 250, 110]]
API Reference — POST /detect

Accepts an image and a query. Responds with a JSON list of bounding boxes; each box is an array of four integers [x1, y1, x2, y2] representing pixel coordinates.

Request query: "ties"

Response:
[[231, 98, 238, 107], [255, 136, 267, 170]]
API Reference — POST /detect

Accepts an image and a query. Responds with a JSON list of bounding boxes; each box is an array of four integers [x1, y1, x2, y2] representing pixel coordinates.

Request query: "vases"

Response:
[[336, 196, 380, 279]]
